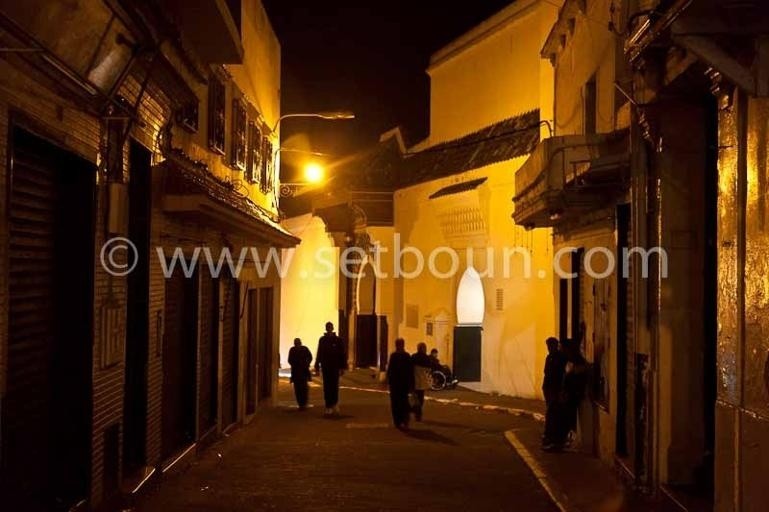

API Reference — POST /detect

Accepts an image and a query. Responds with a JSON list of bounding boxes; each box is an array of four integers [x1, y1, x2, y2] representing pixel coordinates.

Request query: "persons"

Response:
[[540, 336, 589, 453], [410, 342, 436, 422], [384, 336, 415, 431], [288, 337, 314, 414], [313, 322, 349, 419], [430, 347, 461, 388]]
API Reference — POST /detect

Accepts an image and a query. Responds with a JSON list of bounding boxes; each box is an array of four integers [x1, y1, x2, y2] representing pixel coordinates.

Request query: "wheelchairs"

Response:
[[426, 359, 459, 390]]
